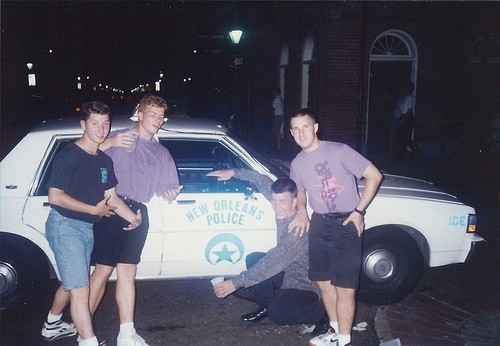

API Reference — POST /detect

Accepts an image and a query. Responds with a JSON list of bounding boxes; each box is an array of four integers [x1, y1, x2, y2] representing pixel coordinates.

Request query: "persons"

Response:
[[87, 95, 184, 346], [389, 83, 426, 162], [271, 88, 285, 151], [286, 108, 383, 346], [205, 168, 331, 336], [41, 102, 143, 346]]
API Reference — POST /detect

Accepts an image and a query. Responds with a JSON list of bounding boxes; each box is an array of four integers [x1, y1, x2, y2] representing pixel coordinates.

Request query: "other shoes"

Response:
[[40, 317, 77, 341], [309, 325, 352, 346], [242, 308, 268, 322], [117, 331, 151, 346]]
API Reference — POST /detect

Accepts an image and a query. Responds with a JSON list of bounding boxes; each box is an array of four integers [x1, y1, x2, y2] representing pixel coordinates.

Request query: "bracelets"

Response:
[[354, 208, 366, 216]]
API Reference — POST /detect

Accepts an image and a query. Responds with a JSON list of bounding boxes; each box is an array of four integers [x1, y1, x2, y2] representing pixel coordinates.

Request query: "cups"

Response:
[[125, 131, 140, 152], [211, 277, 224, 297]]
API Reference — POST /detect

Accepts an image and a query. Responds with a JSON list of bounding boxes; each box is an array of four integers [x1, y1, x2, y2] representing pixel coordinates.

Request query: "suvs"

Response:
[[0, 115, 487, 319]]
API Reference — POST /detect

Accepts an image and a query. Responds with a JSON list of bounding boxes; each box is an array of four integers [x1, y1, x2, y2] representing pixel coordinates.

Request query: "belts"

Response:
[[119, 195, 136, 208]]
